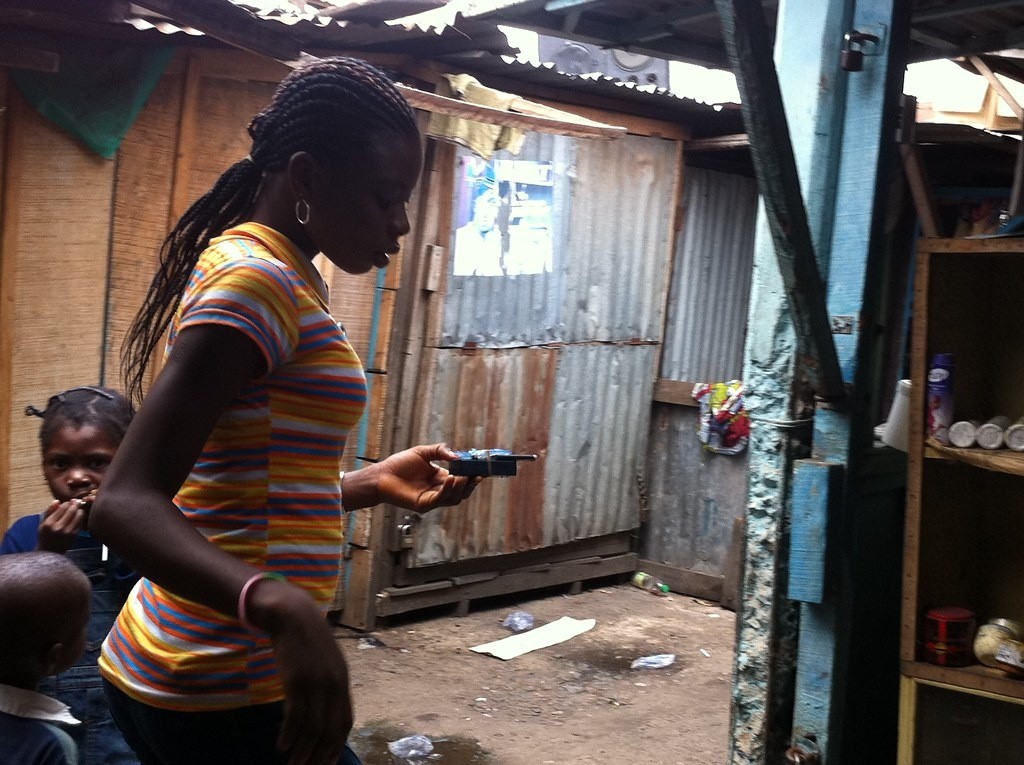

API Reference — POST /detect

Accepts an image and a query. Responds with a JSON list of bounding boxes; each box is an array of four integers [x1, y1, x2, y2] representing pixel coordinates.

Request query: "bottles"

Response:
[[627, 571, 672, 598]]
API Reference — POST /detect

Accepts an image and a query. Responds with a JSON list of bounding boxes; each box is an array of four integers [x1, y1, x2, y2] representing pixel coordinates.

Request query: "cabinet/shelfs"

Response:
[[896, 127, 1024, 765]]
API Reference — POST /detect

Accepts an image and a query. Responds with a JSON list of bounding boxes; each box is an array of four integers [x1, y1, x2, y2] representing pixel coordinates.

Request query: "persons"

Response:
[[0, 385, 143, 765], [88, 56, 483, 765], [0, 551, 94, 765]]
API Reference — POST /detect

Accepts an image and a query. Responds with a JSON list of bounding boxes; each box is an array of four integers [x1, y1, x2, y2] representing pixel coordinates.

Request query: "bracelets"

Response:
[[239, 572, 287, 634]]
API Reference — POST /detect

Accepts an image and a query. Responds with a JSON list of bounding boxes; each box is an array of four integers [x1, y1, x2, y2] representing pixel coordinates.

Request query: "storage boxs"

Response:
[[915, 72, 1024, 130]]
